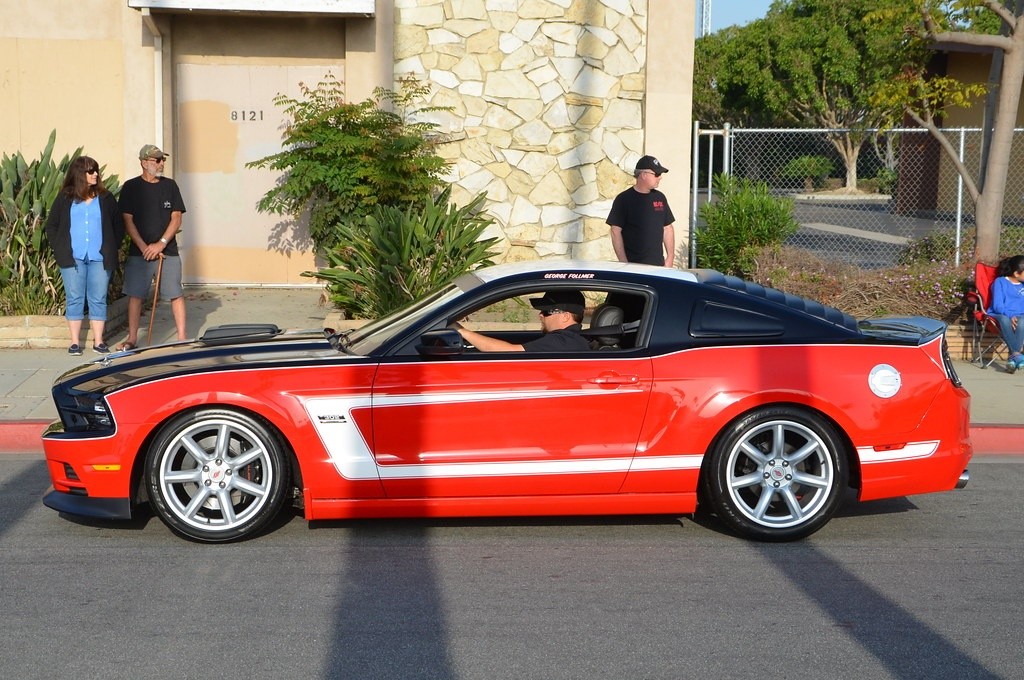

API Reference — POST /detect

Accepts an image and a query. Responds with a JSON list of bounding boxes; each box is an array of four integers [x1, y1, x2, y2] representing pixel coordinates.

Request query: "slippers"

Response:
[[116, 342, 138, 351]]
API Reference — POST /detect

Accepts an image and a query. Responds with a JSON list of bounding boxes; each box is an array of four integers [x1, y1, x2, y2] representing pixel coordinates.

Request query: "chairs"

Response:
[[966, 263, 1010, 367], [586, 305, 618, 352]]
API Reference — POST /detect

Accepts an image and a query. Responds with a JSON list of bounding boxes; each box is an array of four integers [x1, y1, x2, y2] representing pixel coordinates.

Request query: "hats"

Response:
[[636, 155, 669, 173], [528, 290, 585, 314], [139, 144, 170, 160]]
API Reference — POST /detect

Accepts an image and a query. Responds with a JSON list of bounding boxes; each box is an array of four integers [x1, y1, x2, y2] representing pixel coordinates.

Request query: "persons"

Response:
[[445, 288, 593, 353], [115, 145, 187, 352], [605, 155, 676, 268], [986, 255, 1024, 374], [47, 156, 125, 356]]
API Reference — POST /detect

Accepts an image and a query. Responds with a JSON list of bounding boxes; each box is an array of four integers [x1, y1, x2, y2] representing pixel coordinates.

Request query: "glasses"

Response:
[[146, 157, 166, 163], [541, 309, 565, 317], [645, 172, 661, 177], [85, 168, 98, 175]]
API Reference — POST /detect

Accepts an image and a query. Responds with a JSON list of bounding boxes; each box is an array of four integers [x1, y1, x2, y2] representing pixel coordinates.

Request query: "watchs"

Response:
[[159, 237, 167, 245]]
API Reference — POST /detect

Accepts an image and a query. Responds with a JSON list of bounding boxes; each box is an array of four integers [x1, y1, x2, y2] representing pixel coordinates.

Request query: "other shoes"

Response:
[[1014, 354, 1024, 370], [1005, 355, 1017, 374], [68, 344, 83, 356], [93, 343, 111, 354]]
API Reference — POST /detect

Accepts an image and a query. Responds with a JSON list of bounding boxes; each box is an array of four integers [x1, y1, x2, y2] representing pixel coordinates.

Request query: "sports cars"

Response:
[[41, 257, 975, 545]]
